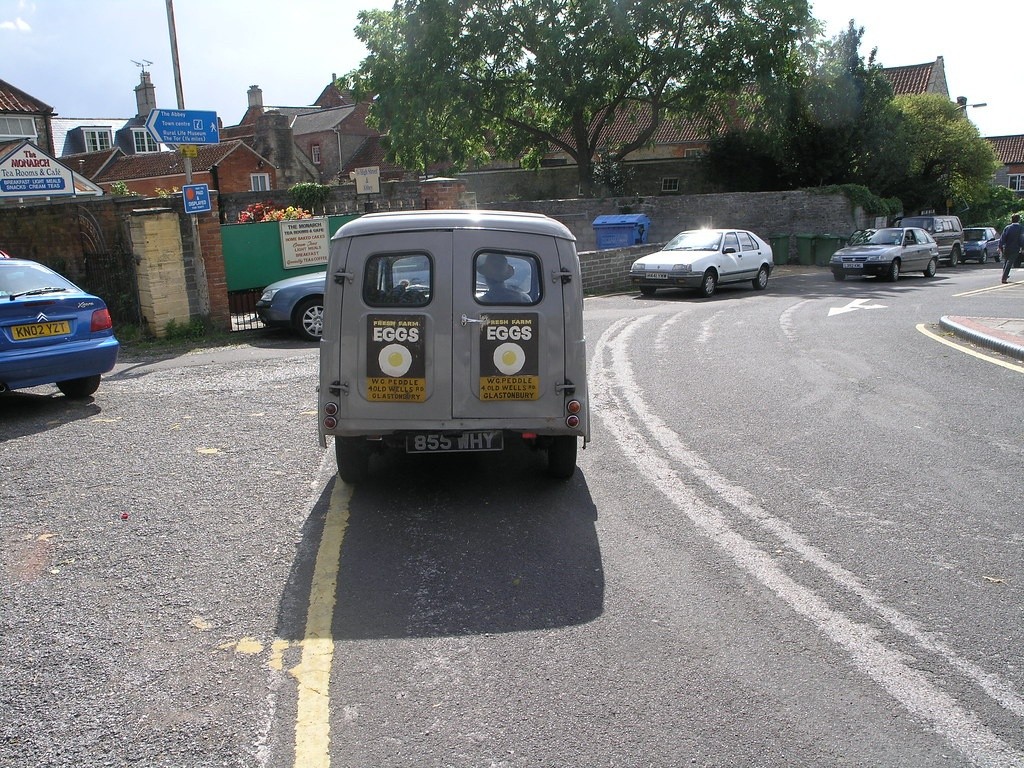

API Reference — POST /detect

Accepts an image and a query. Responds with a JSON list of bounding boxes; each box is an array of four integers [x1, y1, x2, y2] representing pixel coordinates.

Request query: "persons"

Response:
[[474, 252, 533, 303], [998, 213, 1024, 284], [900, 235, 911, 246]]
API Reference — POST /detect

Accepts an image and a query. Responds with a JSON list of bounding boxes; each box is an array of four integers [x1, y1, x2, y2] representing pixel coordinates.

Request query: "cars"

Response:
[[255, 255, 530, 342], [828, 228, 939, 283], [0, 257, 119, 399], [962, 227, 1002, 265], [630, 227, 775, 298]]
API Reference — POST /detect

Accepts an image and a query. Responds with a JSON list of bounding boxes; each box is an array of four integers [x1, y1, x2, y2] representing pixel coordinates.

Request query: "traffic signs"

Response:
[[145, 108, 221, 144]]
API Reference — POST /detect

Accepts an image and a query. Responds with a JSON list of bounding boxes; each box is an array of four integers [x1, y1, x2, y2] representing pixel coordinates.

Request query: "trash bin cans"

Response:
[[591, 212, 651, 250], [769, 233, 790, 265], [817, 232, 841, 266], [794, 233, 817, 265]]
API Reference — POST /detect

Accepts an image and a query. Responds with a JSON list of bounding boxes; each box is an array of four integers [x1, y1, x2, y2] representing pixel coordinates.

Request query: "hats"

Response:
[[477, 253, 514, 281], [1012, 215, 1020, 223]]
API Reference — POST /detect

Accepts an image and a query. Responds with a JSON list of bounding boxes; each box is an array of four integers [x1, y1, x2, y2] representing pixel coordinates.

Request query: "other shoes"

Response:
[[1002, 280, 1008, 284]]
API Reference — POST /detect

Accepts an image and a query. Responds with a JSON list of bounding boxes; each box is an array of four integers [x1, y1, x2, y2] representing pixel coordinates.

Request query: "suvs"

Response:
[[898, 216, 966, 267]]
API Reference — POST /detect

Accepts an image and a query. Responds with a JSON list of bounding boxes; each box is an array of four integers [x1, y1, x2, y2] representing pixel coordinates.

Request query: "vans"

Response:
[[319, 209, 590, 487]]
